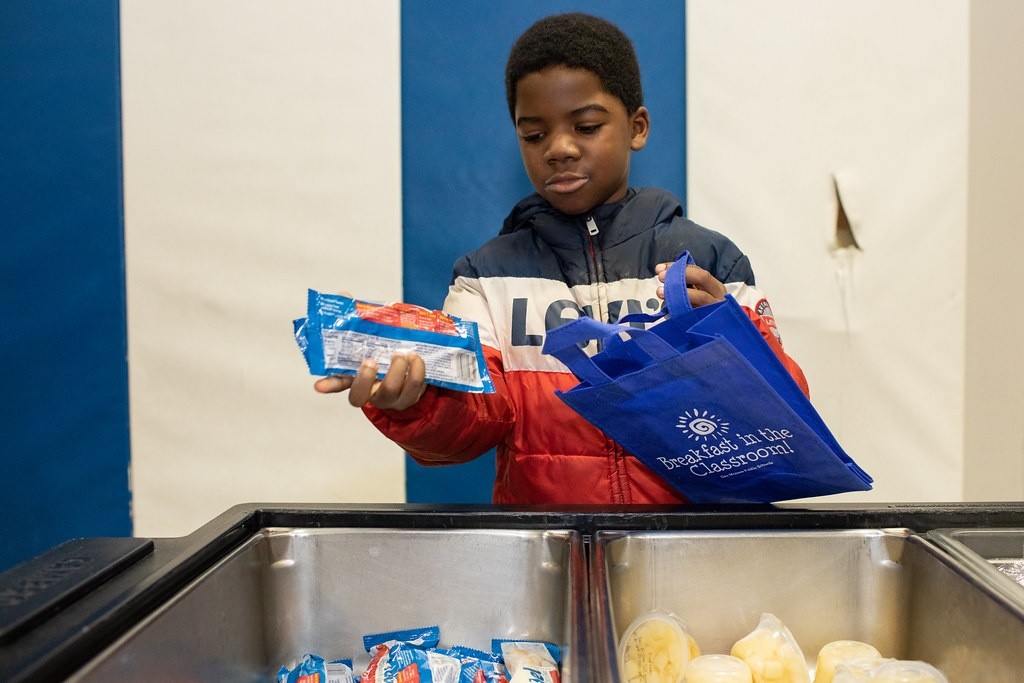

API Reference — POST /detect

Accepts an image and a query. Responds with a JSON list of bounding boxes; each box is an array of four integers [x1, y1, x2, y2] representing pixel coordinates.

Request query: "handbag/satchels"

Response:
[[540, 249, 875, 504]]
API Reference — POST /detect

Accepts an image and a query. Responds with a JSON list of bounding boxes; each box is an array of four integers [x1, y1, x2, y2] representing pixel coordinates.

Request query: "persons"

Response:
[[314, 13, 810, 505]]
